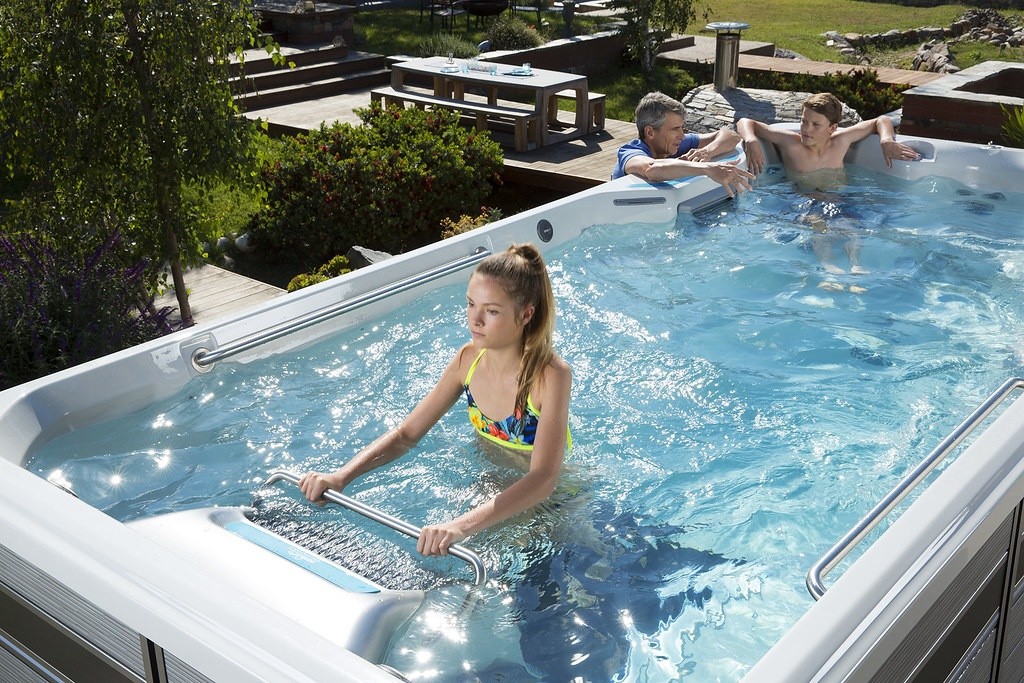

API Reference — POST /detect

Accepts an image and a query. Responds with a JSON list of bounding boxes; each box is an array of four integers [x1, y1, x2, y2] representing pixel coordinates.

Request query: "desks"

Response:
[[389, 52, 589, 148]]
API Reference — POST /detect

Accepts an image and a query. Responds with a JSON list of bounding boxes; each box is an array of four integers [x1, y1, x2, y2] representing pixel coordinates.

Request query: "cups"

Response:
[[461, 59, 497, 76], [523, 63, 531, 75]]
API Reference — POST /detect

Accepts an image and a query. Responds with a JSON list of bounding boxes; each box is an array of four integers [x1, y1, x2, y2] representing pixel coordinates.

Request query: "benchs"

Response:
[[547, 87, 608, 131], [372, 82, 543, 157]]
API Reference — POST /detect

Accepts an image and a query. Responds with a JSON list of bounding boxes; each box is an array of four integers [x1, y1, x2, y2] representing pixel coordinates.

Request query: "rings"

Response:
[[734, 180, 738, 184]]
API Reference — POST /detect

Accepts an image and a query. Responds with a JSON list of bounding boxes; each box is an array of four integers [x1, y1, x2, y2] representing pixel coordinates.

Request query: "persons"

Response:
[[300, 242, 571, 557], [737, 92, 919, 175], [612, 92, 756, 197]]
